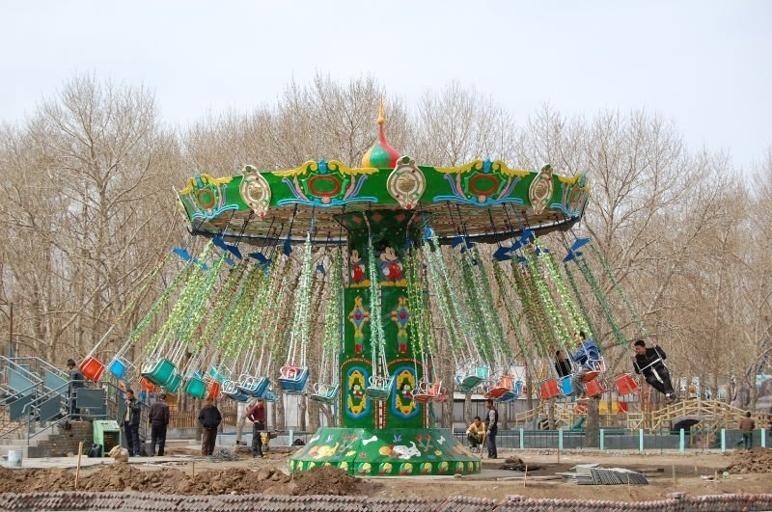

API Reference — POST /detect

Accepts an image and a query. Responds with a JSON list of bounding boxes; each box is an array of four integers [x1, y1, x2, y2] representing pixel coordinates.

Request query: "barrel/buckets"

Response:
[[7, 449, 23, 468]]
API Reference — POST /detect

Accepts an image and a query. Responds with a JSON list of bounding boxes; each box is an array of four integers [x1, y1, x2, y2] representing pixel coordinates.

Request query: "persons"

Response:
[[630, 339, 678, 401], [66, 359, 84, 422], [573, 330, 603, 403], [554, 349, 573, 378], [119, 389, 171, 457], [465, 399, 499, 459], [197, 398, 223, 456], [245, 397, 277, 459], [739, 411, 756, 452]]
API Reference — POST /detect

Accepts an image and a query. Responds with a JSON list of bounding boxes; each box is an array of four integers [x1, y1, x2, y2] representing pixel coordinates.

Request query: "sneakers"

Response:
[[665, 393, 676, 404]]
